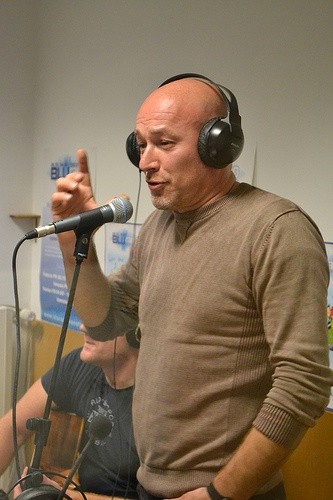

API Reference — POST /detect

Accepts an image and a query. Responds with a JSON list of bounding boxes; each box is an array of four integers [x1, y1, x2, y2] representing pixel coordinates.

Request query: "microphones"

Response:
[[57, 415, 112, 500], [25, 197, 133, 239]]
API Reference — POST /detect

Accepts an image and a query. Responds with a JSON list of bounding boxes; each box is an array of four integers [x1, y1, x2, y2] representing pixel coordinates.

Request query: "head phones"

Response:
[[126, 73, 244, 172], [126, 326, 142, 348]]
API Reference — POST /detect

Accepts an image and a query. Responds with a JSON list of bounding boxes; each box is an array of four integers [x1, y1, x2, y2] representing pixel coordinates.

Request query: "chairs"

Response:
[[30, 409, 83, 478]]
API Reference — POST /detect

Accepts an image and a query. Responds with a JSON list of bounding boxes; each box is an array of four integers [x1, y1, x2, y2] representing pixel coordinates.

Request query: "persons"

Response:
[[0, 323, 138, 500], [50, 73, 333, 500]]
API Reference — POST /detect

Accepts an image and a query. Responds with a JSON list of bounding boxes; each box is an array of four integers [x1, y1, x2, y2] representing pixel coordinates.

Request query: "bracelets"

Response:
[[207, 482, 234, 500]]
